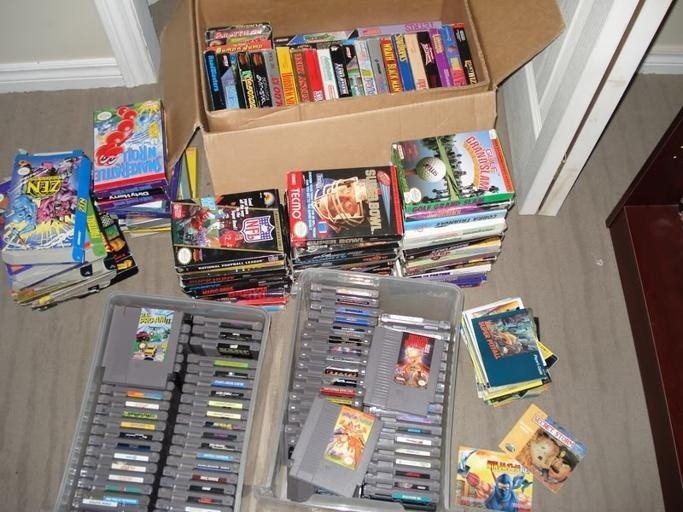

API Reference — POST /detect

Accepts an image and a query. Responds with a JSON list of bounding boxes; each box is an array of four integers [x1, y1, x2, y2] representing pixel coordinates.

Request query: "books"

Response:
[[456, 297, 559, 408], [0, 20, 516, 313], [455, 444, 535, 511], [496, 405, 590, 492]]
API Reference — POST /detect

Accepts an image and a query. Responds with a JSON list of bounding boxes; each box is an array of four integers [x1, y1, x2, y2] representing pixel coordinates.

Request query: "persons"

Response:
[[539, 445, 578, 486]]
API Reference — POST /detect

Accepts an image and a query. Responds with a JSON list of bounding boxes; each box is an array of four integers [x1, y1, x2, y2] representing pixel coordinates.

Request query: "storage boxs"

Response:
[[193, 2, 492, 131], [160, 1, 567, 207]]
